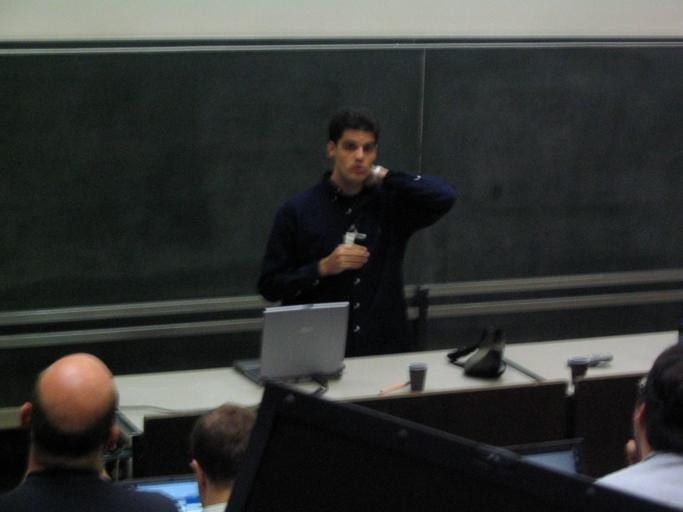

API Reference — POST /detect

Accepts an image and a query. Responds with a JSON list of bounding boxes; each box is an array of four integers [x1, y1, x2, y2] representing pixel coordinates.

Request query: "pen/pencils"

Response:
[[379, 380, 411, 394]]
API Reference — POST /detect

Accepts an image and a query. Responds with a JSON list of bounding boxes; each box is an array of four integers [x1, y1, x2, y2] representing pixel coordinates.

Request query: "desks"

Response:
[[107, 344, 538, 431], [501, 331, 682, 383]]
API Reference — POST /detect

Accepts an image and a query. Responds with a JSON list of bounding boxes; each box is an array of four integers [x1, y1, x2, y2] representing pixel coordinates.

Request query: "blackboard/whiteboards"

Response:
[[0, 34, 683, 350]]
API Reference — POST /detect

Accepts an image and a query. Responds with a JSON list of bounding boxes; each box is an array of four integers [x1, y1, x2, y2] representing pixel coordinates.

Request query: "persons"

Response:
[[188, 402, 258, 512], [0, 351, 180, 512], [594, 343, 683, 512], [258, 106, 456, 357]]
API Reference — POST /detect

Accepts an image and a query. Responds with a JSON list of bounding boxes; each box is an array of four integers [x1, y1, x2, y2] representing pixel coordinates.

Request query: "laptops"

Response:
[[231, 301, 349, 386]]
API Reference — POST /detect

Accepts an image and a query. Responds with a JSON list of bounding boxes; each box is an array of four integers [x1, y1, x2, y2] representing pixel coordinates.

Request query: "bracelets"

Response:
[[371, 165, 382, 185]]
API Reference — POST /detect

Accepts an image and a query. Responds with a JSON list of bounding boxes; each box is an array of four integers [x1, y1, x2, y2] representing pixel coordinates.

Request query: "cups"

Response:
[[567, 356, 588, 383], [409, 363, 428, 389]]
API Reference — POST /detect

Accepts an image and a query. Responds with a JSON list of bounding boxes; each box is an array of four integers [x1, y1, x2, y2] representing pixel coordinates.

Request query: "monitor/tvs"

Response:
[[508, 437, 586, 477], [125, 475, 206, 511]]
